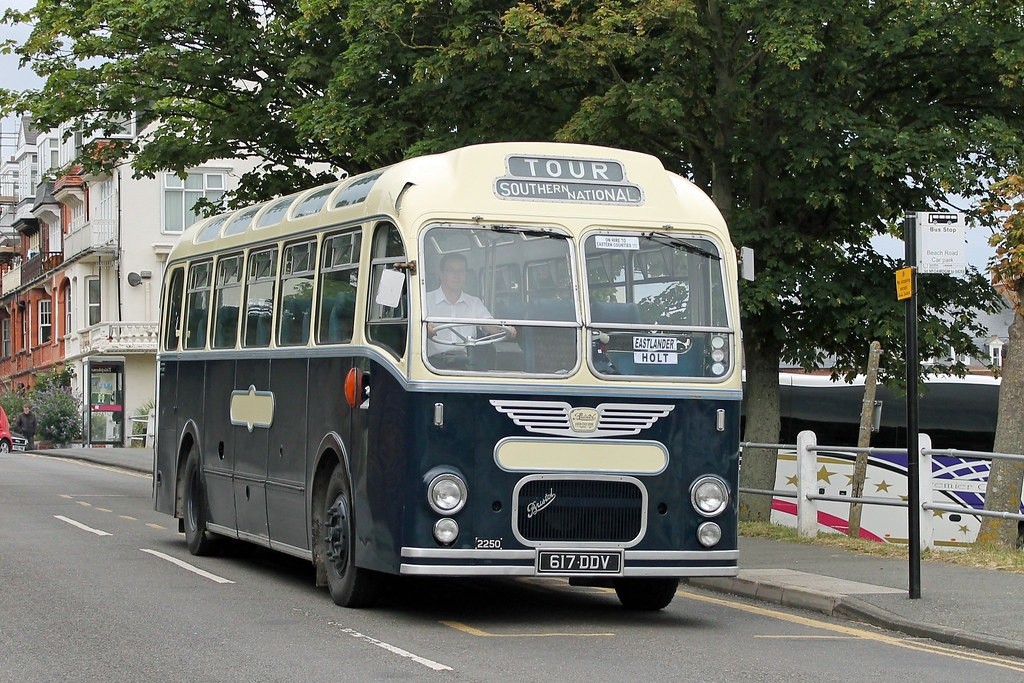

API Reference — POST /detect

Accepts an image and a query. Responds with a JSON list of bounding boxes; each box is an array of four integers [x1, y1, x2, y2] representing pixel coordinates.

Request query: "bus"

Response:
[[152, 140, 754, 614], [738, 373, 1024, 551]]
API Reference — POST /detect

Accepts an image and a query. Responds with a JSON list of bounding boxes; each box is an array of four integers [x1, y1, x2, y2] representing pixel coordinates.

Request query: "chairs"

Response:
[[282, 309, 309, 345], [246, 304, 271, 346], [328, 291, 354, 343], [197, 318, 206, 347], [216, 305, 238, 347], [492, 299, 642, 372], [188, 308, 202, 348], [302, 297, 334, 344]]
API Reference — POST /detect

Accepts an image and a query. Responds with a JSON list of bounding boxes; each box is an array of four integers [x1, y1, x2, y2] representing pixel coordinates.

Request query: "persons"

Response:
[[426, 252, 517, 368], [14, 404, 37, 449]]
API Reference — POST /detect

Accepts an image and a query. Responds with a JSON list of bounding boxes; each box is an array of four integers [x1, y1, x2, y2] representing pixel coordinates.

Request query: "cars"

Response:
[[0, 406, 13, 455], [10, 430, 28, 453]]
